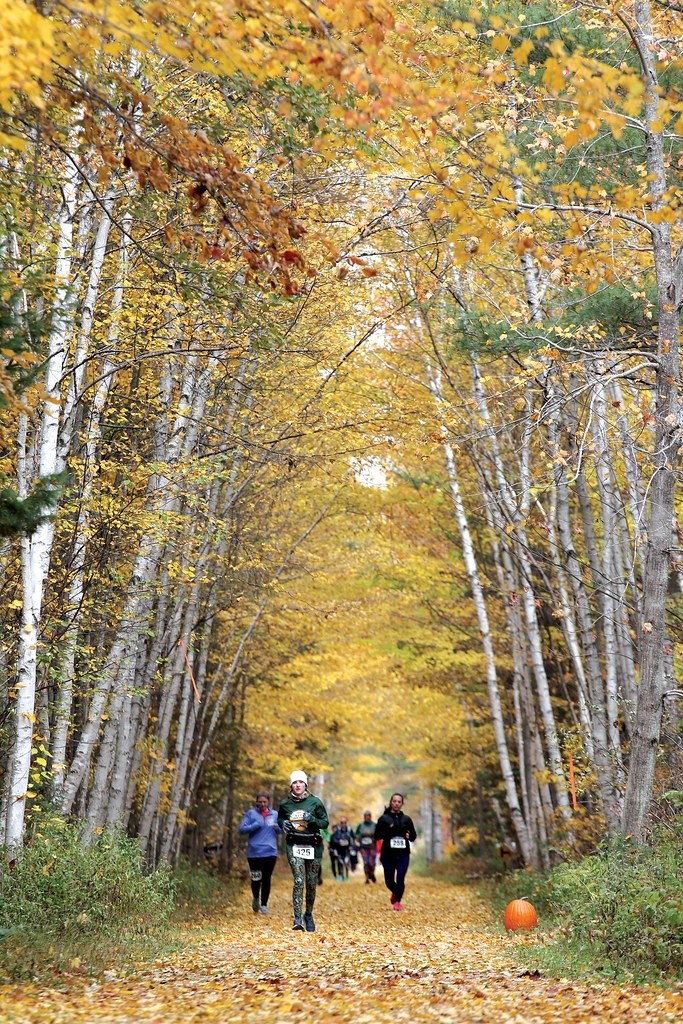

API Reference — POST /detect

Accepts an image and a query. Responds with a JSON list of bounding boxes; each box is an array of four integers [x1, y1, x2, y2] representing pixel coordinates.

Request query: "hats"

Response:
[[289, 771, 307, 786]]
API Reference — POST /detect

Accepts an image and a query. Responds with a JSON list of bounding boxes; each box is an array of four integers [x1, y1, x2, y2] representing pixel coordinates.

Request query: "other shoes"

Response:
[[391, 894, 396, 904], [394, 902, 402, 910], [260, 906, 268, 913], [252, 899, 259, 911], [372, 877, 376, 882]]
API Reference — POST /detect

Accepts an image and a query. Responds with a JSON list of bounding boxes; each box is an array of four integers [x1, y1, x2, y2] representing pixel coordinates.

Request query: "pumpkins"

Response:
[[505, 896, 537, 932]]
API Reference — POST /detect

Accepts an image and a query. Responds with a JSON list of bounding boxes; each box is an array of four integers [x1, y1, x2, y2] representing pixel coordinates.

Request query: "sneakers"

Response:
[[303, 914, 315, 931], [292, 919, 304, 930]]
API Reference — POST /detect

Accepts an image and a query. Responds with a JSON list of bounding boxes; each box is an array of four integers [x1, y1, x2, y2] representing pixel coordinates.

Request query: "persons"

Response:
[[277, 770, 328, 930], [311, 811, 381, 884], [239, 788, 282, 913], [375, 792, 417, 910]]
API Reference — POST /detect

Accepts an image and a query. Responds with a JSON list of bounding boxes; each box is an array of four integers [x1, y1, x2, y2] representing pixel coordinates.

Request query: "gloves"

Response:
[[283, 820, 292, 833], [303, 813, 311, 821]]
